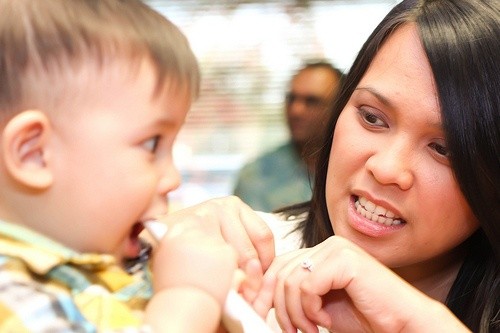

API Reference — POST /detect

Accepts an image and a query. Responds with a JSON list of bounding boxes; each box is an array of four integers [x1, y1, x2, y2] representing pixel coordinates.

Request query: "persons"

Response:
[[156, 0, 500, 333], [0, 0, 237, 333], [232, 63, 346, 213]]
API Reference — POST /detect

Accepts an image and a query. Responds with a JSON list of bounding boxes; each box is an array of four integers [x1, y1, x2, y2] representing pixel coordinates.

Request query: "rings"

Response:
[[299, 257, 314, 272]]
